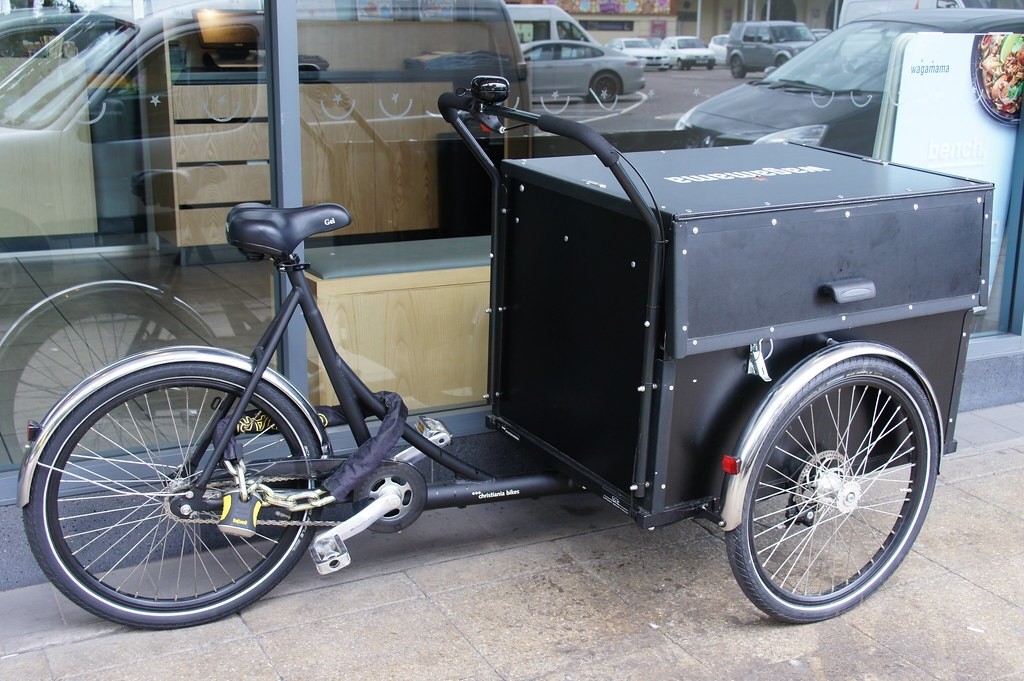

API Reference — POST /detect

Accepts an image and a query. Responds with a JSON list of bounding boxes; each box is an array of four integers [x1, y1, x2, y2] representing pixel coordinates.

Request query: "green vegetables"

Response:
[[1010, 80, 1024, 99]]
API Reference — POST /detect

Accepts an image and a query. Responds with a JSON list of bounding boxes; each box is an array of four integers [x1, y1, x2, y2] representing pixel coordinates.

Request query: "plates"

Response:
[[970, 34, 1021, 129]]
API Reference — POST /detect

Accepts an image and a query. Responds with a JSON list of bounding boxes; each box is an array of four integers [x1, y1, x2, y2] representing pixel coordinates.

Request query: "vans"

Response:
[[2, 0, 536, 243], [835, 0, 1024, 68], [506, 3, 600, 47], [725, 20, 818, 79]]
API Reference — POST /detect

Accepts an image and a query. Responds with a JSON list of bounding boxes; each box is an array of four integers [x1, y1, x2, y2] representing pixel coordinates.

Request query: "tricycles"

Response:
[[11, 70, 998, 632], [0, 164, 269, 469]]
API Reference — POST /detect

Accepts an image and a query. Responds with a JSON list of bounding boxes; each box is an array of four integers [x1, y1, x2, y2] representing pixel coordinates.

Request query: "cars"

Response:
[[673, 7, 1024, 159], [1, 6, 136, 107], [520, 39, 646, 103], [647, 33, 730, 70], [604, 37, 670, 72]]
